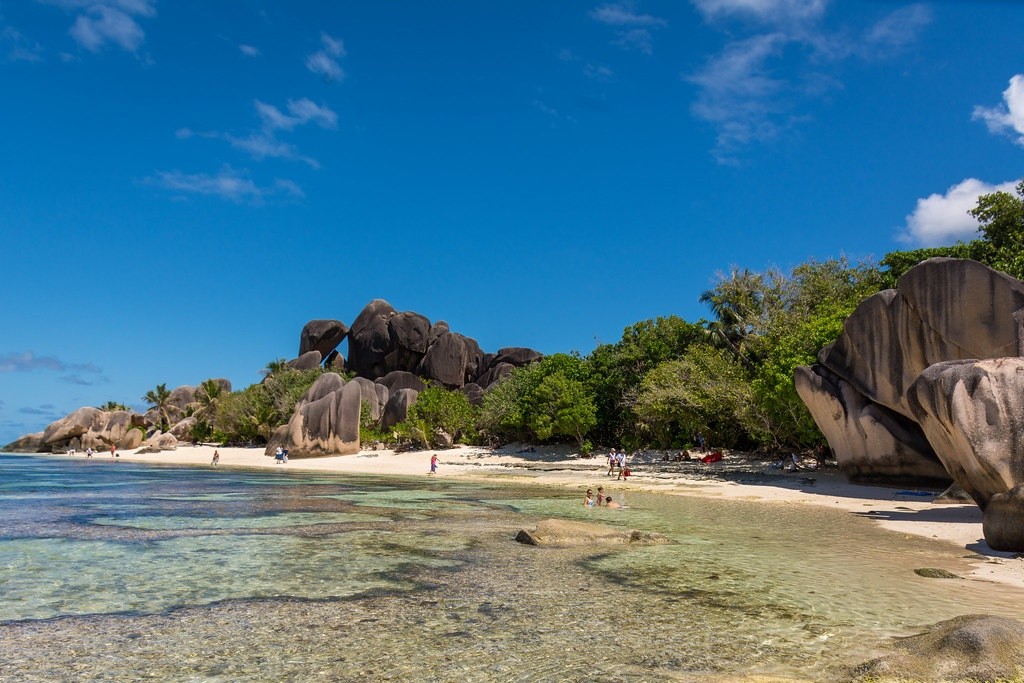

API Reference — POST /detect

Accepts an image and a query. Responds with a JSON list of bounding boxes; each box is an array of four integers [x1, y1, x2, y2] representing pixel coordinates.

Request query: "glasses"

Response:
[[588, 492, 592, 494]]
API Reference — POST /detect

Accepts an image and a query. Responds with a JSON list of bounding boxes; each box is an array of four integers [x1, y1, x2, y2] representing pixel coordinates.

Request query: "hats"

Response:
[[611, 448, 616, 451], [620, 449, 625, 452]]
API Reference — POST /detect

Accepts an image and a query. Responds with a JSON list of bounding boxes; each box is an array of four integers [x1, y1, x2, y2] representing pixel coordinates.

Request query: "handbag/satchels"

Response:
[[624, 468, 630, 476], [617, 462, 620, 467]]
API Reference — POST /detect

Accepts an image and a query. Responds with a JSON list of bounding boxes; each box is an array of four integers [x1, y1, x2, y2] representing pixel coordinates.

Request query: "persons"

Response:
[[607, 448, 617, 477], [430, 454, 439, 473], [111, 443, 116, 457], [87, 447, 92, 458], [606, 497, 618, 506], [616, 449, 627, 480], [596, 486, 604, 505], [663, 449, 690, 461], [584, 489, 594, 506], [212, 450, 219, 466], [275, 443, 289, 464]]
